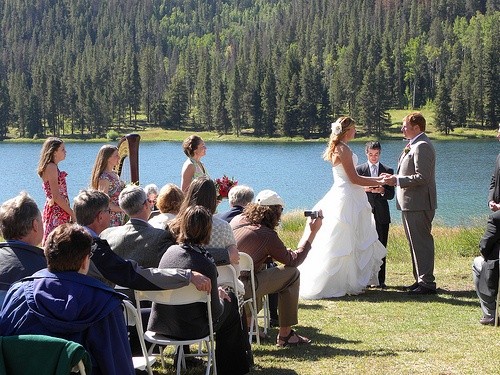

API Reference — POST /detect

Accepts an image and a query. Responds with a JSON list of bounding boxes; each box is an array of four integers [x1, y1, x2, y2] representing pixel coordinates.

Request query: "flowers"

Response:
[[405, 143, 411, 153], [120, 180, 139, 221], [331, 121, 342, 134], [214, 175, 238, 200], [61, 170, 68, 178]]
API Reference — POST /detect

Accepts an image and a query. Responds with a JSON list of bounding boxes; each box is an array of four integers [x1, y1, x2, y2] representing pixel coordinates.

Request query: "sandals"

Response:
[[247, 325, 271, 339], [276, 330, 311, 348]]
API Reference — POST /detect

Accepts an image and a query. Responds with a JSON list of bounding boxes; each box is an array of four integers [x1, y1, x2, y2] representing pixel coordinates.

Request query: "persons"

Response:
[[91, 145, 127, 228], [356, 140, 394, 291], [38, 137, 74, 247], [181, 134, 207, 191], [296, 116, 388, 302], [385, 114, 436, 295], [471, 154, 500, 328], [0, 177, 323, 375]]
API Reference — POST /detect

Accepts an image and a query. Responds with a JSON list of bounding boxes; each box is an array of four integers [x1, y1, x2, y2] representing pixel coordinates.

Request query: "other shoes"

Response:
[[478, 313, 499, 325], [407, 285, 437, 295], [403, 282, 419, 290]]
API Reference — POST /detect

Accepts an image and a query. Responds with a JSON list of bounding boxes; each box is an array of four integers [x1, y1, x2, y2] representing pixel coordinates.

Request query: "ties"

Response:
[[372, 164, 378, 177]]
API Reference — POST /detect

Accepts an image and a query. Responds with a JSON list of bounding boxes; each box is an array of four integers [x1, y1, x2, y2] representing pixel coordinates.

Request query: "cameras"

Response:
[[304, 210, 318, 219]]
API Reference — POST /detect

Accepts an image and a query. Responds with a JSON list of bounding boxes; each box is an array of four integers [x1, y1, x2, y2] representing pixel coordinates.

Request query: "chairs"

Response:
[[0, 251, 270, 375]]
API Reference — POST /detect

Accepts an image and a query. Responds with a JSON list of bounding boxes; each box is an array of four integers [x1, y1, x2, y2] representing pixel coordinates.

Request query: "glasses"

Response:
[[103, 208, 111, 214], [88, 251, 95, 259]]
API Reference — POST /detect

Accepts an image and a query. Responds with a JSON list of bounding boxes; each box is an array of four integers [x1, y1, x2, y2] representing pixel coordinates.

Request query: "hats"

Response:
[[255, 190, 283, 205]]
